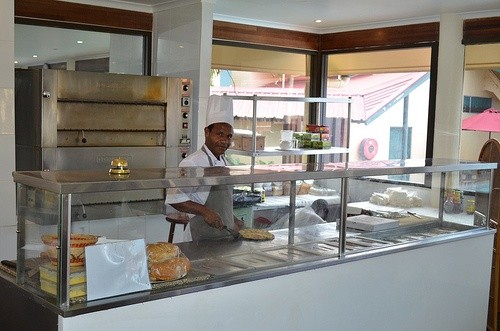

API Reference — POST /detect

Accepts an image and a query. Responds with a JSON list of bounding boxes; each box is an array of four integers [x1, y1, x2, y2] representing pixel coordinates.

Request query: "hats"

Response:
[[206, 94, 233, 128]]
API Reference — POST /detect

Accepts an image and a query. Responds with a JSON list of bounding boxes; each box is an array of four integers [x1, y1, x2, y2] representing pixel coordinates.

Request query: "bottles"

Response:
[[261, 187, 265, 202]]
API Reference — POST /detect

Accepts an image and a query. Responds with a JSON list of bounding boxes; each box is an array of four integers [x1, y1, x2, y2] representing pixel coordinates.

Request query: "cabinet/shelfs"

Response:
[[214, 95, 352, 223], [0, 162, 500, 331]]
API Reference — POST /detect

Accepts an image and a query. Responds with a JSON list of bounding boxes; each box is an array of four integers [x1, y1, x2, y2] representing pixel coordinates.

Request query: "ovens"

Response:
[[14, 67, 193, 207]]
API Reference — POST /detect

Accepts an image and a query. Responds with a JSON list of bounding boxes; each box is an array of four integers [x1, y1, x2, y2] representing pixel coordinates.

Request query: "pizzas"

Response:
[[237, 229, 273, 239]]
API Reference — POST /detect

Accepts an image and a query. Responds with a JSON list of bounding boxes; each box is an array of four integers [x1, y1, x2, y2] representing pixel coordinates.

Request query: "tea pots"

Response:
[[279, 141, 292, 149]]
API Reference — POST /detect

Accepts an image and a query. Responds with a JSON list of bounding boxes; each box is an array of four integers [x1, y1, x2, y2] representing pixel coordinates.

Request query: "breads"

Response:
[[147, 242, 191, 281]]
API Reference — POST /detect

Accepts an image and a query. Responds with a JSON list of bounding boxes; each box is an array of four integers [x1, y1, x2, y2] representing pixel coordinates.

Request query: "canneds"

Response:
[[466, 199, 476, 215]]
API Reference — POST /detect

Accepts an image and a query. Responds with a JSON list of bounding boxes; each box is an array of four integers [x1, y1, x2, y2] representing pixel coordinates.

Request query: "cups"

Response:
[[280, 130, 293, 150]]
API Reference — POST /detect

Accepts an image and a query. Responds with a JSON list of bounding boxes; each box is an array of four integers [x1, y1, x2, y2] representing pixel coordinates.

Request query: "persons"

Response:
[[267, 199, 329, 231], [164, 94, 248, 241]]
